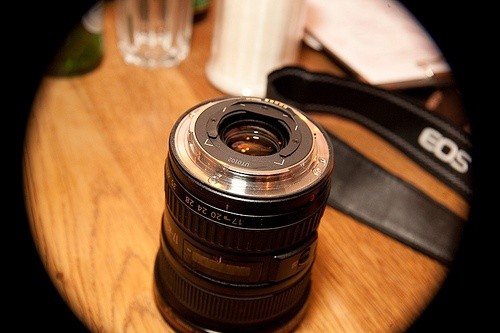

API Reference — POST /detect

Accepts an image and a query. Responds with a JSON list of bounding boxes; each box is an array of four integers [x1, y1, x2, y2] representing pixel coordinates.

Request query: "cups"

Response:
[[114, 1, 194, 66], [204, 0, 311, 99]]
